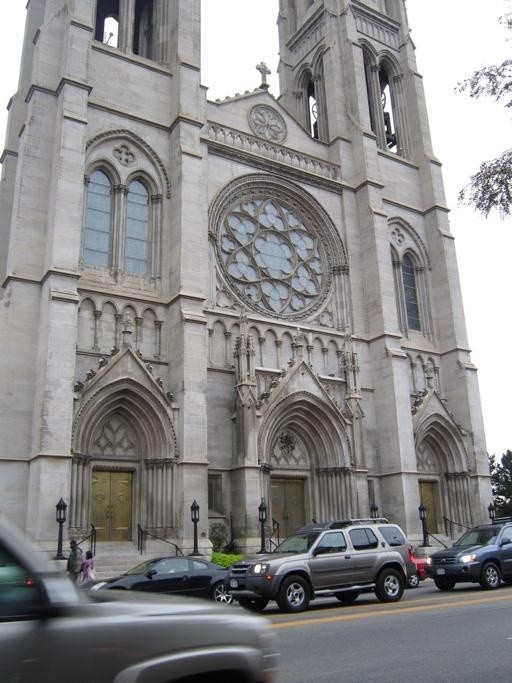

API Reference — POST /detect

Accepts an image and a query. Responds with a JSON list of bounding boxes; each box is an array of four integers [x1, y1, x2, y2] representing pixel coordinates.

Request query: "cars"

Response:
[[408, 544, 431, 588]]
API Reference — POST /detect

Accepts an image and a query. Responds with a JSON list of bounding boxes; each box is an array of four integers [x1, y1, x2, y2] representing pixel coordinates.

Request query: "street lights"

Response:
[[487, 502, 495, 524], [418, 503, 432, 546], [257, 497, 268, 553], [370, 500, 379, 522], [189, 499, 205, 555], [54, 496, 69, 559]]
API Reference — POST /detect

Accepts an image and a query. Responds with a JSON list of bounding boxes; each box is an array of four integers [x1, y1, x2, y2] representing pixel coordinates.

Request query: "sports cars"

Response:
[[80, 553, 236, 606]]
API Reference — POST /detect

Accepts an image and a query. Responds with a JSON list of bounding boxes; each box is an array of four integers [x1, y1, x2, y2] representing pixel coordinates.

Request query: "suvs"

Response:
[[225, 517, 419, 613], [426, 516, 512, 591], [1, 513, 278, 681]]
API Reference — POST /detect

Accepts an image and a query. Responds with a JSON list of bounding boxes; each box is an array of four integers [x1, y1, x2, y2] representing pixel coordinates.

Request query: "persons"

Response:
[[81, 551, 97, 584], [66, 538, 83, 582]]
